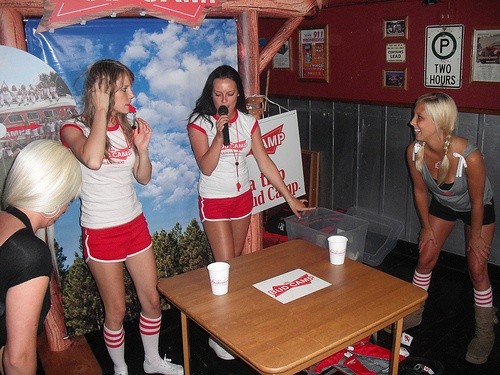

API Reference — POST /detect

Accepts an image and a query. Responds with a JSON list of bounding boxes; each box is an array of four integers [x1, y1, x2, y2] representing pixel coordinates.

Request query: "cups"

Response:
[[207, 261, 230, 295], [327, 235, 348, 265]]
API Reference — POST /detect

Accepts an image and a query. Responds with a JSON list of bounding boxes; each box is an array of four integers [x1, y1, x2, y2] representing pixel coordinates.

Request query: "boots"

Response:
[[385, 301, 425, 334], [465, 306, 496, 364]]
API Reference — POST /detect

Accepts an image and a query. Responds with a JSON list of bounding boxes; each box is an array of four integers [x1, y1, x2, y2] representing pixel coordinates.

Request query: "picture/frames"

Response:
[[271, 15, 500, 89]]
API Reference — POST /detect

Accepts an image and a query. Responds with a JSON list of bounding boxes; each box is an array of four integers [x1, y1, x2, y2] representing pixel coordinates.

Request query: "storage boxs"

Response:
[[285, 206, 369, 264]]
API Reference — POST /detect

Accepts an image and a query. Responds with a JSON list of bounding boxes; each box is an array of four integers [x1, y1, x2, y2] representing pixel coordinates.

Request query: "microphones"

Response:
[[218, 105, 230, 145]]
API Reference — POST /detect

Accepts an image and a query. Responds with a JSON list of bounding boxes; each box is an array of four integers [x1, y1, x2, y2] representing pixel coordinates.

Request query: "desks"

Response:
[[156, 240, 428, 375]]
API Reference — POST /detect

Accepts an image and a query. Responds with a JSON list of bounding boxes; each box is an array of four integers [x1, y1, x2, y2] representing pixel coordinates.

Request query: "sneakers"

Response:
[[209, 337, 235, 360], [143, 358, 183, 375], [115, 366, 127, 375]]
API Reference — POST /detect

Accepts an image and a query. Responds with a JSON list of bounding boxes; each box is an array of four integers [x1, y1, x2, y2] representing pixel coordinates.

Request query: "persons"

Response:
[[0, 138, 82, 375], [383, 91, 496, 364], [186, 65, 316, 263], [0, 80, 79, 157], [59, 59, 186, 375]]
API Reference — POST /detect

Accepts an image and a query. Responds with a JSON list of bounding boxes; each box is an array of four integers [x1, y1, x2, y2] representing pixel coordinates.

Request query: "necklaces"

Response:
[[211, 110, 242, 192], [428, 144, 443, 170]]
